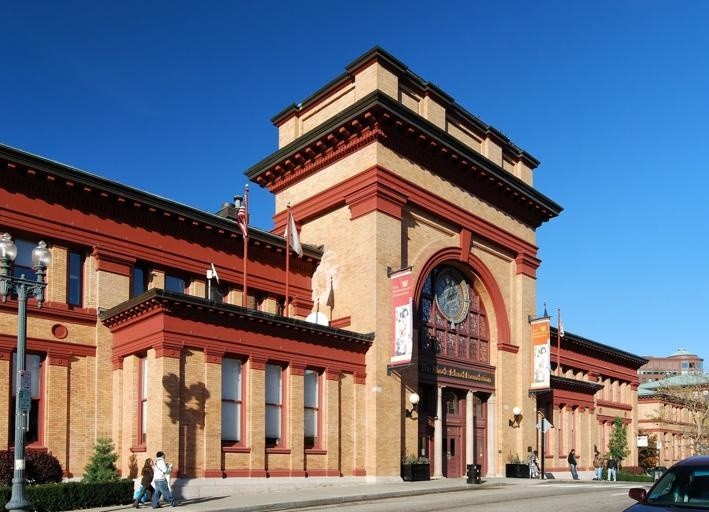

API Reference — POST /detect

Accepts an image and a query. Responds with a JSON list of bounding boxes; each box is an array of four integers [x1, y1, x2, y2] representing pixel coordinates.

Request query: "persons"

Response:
[[537, 347, 547, 382], [398, 309, 409, 353], [607, 456, 617, 481], [133, 451, 176, 509], [568, 449, 580, 480], [593, 453, 604, 480]]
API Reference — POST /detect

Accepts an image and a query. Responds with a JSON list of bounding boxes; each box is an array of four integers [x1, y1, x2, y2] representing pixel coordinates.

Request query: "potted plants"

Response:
[[505, 452, 531, 478], [401, 452, 431, 481]]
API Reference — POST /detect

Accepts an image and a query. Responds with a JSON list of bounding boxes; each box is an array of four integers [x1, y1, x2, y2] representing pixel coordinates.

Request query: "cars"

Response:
[[622, 455, 709, 512]]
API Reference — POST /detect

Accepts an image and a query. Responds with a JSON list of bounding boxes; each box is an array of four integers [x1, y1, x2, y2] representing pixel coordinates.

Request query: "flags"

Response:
[[237, 194, 247, 239], [284, 210, 303, 259]]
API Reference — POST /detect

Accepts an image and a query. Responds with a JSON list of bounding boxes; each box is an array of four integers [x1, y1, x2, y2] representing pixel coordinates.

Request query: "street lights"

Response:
[[0, 231, 53, 512]]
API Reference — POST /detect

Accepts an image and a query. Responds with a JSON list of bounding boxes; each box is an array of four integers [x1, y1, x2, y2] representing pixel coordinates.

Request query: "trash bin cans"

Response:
[[467, 464, 482, 484]]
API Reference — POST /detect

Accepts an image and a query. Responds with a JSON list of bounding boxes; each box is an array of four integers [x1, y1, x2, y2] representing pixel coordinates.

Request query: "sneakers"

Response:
[[132, 501, 178, 508]]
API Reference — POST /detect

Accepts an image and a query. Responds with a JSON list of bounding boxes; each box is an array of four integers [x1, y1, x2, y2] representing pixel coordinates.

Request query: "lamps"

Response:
[[405, 391, 420, 417], [508, 406, 521, 427]]
